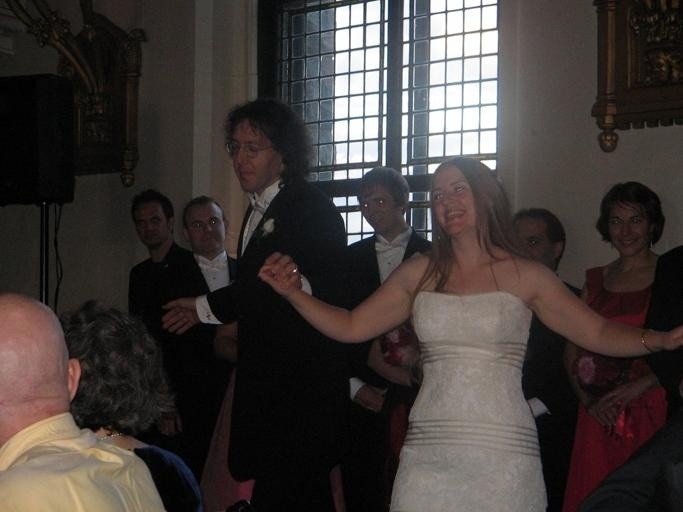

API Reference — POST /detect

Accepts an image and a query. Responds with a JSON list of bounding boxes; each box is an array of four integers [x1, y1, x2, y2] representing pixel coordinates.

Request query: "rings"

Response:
[[291, 264, 297, 272]]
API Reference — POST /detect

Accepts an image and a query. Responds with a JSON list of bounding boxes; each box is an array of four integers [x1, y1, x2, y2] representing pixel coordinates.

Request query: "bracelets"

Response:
[[640, 329, 660, 354]]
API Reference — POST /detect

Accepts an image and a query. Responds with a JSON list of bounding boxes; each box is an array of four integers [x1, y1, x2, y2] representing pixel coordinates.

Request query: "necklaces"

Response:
[[96, 430, 124, 440]]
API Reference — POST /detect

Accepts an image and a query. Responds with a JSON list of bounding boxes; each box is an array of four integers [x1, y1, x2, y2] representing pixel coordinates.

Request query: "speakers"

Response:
[[1, 72, 76, 209]]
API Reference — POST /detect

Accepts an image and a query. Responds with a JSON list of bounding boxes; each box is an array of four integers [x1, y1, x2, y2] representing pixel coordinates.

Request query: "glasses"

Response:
[[190, 218, 221, 231], [225, 142, 274, 159]]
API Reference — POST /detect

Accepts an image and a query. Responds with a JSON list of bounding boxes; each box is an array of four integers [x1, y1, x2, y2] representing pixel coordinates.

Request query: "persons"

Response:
[[56, 299, 203, 512], [258, 158, 682, 512], [158, 195, 238, 428], [160, 98, 348, 510], [127, 189, 201, 322], [561, 180, 668, 512], [644, 243, 682, 421], [510, 207, 579, 511], [0, 292, 167, 512], [333, 166, 432, 512], [578, 419, 682, 511]]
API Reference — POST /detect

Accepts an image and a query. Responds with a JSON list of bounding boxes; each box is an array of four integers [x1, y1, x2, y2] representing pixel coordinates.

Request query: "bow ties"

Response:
[[199, 255, 226, 271], [247, 192, 266, 215], [375, 241, 407, 253]]
